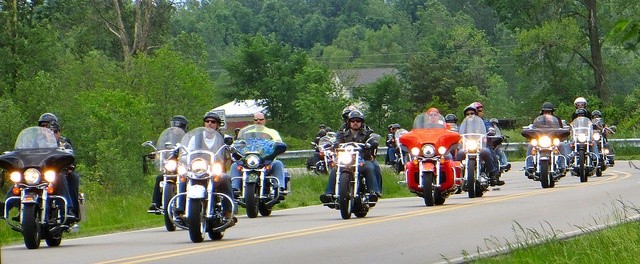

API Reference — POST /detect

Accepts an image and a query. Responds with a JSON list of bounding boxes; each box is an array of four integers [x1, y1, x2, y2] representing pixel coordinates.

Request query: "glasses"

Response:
[[429, 113, 437, 116], [253, 117, 265, 121], [204, 120, 217, 123], [465, 111, 476, 115], [350, 119, 362, 122]]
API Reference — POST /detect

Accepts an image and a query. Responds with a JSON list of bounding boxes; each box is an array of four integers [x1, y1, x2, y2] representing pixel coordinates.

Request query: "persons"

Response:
[[389, 123, 402, 164], [386, 125, 394, 148], [591, 110, 607, 147], [443, 113, 458, 125], [468, 102, 494, 148], [320, 124, 325, 129], [525, 102, 569, 171], [230, 112, 285, 196], [235, 127, 240, 139], [1, 113, 80, 224], [324, 127, 332, 132], [314, 129, 327, 167], [422, 107, 454, 162], [149, 114, 189, 211], [573, 108, 601, 161], [176, 112, 233, 223], [458, 106, 505, 186], [335, 106, 382, 197], [490, 118, 512, 173], [571, 97, 591, 119], [320, 110, 380, 209]]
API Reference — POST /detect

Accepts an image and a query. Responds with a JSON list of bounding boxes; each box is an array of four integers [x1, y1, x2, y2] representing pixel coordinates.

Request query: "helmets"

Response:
[[591, 109, 602, 118], [542, 102, 554, 111], [463, 105, 478, 116], [341, 105, 358, 121], [38, 112, 58, 126], [319, 128, 326, 135], [469, 102, 482, 108], [444, 113, 457, 123], [348, 109, 365, 123], [489, 117, 499, 123], [325, 126, 332, 131], [46, 120, 61, 132], [319, 123, 326, 127], [170, 114, 188, 132], [202, 111, 220, 122], [574, 96, 587, 106], [392, 123, 401, 128]]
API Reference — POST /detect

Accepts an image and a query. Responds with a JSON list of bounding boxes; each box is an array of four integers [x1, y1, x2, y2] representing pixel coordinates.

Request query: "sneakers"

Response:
[[320, 193, 336, 208], [504, 163, 511, 173], [147, 202, 164, 214], [490, 177, 505, 186], [368, 192, 378, 207]]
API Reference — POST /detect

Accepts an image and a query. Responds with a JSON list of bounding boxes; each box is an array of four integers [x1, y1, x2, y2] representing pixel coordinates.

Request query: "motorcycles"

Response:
[[304, 140, 331, 176], [561, 115, 600, 181], [0, 126, 85, 248], [521, 115, 570, 187], [592, 117, 617, 176], [321, 133, 382, 219], [165, 126, 237, 243], [230, 124, 290, 217], [141, 126, 185, 231], [399, 112, 462, 205], [493, 124, 511, 172], [458, 114, 496, 198], [387, 128, 408, 172]]
